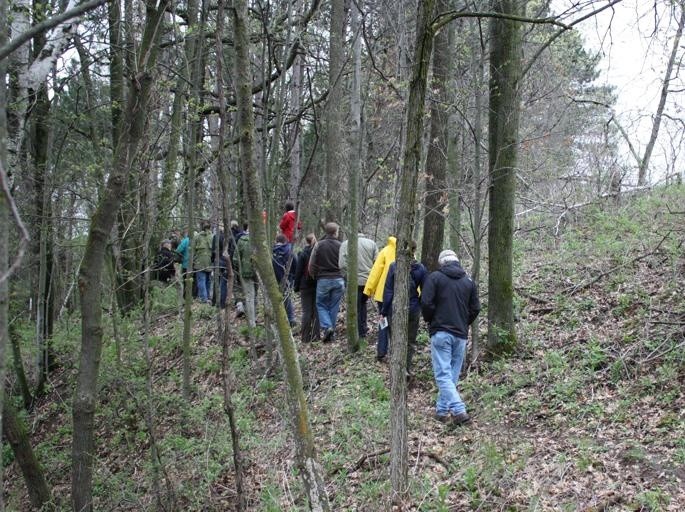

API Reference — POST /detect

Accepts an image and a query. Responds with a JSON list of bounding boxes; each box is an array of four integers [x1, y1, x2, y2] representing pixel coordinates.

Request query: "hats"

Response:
[[438, 249, 457, 260], [161, 239, 172, 245]]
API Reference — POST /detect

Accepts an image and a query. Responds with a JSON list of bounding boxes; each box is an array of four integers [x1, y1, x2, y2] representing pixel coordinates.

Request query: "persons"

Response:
[[338, 233, 379, 338], [382, 239, 426, 381], [157, 220, 259, 319], [273, 235, 299, 328], [307, 222, 342, 343], [293, 232, 318, 343], [279, 202, 301, 244], [419, 249, 481, 426], [362, 235, 423, 360]]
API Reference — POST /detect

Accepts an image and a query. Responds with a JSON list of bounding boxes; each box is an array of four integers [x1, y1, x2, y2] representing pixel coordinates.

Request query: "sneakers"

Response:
[[234, 307, 245, 319], [312, 327, 336, 343], [438, 412, 470, 427], [292, 326, 301, 337], [406, 372, 417, 390], [375, 355, 388, 362]]
